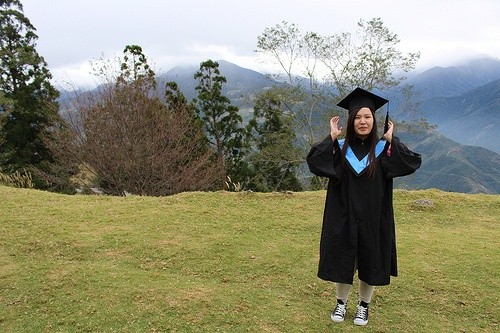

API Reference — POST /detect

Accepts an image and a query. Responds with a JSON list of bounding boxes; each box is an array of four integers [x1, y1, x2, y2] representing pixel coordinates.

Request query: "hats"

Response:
[[336, 87, 390, 134]]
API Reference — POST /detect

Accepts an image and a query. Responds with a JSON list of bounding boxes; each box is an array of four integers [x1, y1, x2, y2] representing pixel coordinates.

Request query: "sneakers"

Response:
[[331, 302, 347, 322], [354, 305, 368, 325]]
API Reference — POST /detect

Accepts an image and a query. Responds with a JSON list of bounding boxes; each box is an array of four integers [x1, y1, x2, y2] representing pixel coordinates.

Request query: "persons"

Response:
[[306, 87, 422, 326]]
